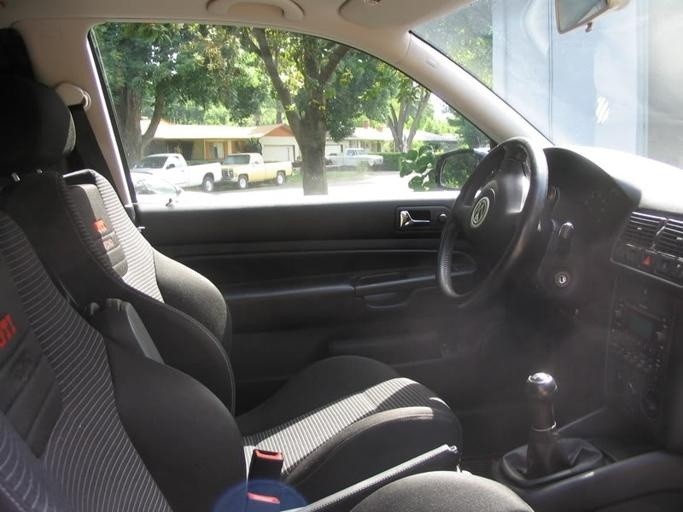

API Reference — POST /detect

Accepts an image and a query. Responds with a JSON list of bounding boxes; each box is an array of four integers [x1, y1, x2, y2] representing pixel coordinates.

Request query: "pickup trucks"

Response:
[[219, 154, 294, 189], [328, 148, 383, 167], [132, 153, 223, 194]]
